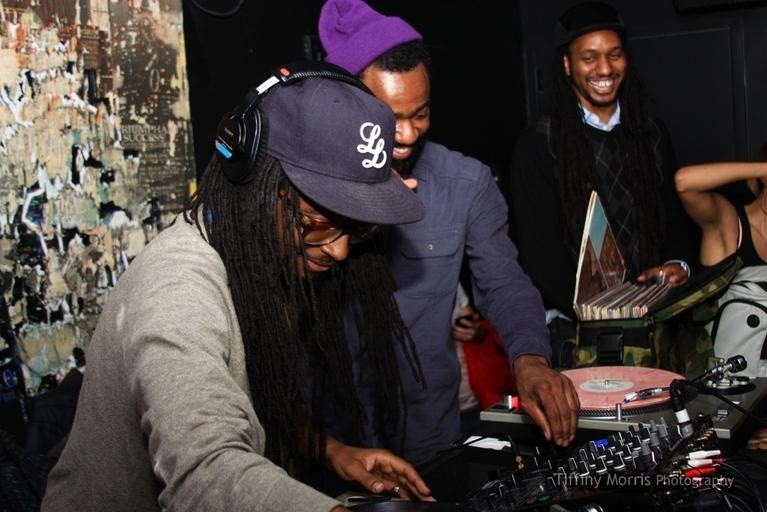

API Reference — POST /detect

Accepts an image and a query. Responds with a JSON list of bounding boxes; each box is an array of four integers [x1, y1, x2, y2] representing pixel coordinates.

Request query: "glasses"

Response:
[[286, 197, 381, 247]]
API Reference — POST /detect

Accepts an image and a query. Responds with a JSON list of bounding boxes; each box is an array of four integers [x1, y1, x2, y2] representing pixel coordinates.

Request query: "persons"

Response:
[[448, 277, 486, 435], [312, 0, 584, 490], [673, 161, 766, 394], [479, 4, 686, 381], [34, 56, 444, 509]]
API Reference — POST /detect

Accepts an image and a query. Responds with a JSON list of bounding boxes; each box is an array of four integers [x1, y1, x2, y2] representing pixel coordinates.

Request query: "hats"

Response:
[[257, 75, 426, 226], [316, 1, 421, 76], [551, 10, 626, 63]]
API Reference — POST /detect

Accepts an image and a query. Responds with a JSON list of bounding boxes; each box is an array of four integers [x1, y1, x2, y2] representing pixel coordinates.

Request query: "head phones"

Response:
[[212, 67, 376, 186]]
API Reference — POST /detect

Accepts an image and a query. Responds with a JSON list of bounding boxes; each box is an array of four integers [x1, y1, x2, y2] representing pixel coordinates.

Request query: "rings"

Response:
[[392, 486, 401, 498]]
